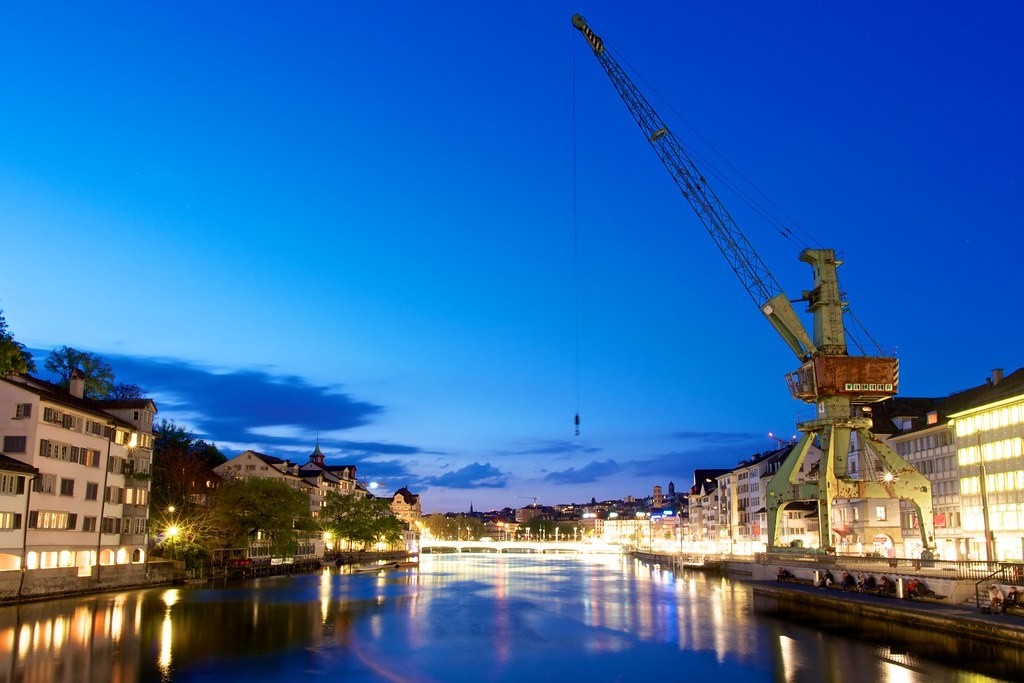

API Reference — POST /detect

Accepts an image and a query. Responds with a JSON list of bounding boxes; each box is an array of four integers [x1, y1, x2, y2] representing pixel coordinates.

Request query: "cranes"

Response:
[[572, 10, 942, 568], [514, 495, 540, 508]]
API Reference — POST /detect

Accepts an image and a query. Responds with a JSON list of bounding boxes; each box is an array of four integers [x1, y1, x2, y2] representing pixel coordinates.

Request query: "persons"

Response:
[[777, 567, 794, 582], [813, 569, 927, 602], [987, 585, 1020, 617]]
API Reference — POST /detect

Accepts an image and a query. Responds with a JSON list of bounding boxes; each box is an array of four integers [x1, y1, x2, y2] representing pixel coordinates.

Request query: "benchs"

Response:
[[994, 605, 1024, 616], [784, 577, 951, 604]]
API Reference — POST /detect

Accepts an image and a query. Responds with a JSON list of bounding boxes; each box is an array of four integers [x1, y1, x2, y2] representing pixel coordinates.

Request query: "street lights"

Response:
[[168, 528, 178, 560], [168, 505, 176, 528], [324, 532, 331, 554]]
